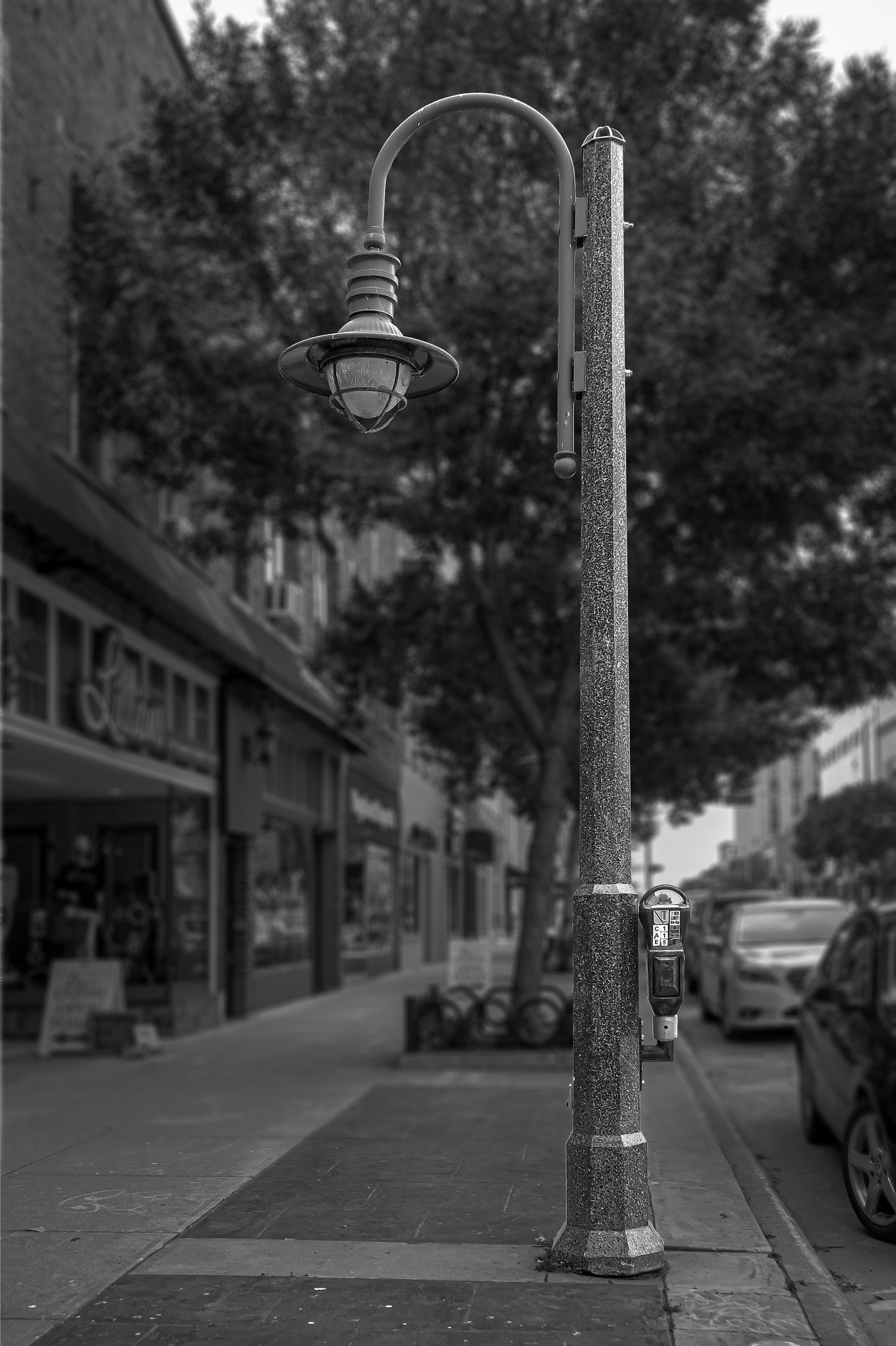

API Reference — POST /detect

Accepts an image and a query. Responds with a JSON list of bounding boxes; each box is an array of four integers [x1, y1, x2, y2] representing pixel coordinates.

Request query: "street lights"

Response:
[[277, 87, 687, 1276]]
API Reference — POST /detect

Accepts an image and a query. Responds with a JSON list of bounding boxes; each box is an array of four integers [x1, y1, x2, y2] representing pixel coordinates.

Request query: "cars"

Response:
[[684, 890, 796, 991], [790, 898, 896, 1239], [698, 898, 850, 1044]]
